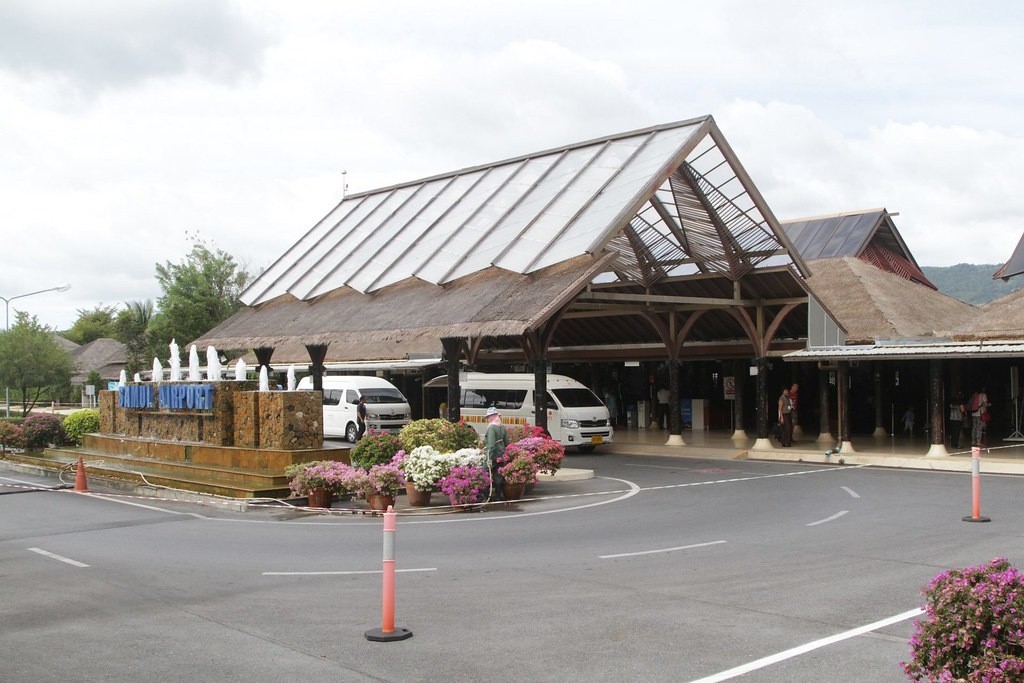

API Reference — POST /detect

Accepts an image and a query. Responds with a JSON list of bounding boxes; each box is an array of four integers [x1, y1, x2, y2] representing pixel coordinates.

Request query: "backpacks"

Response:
[[966, 391, 983, 412]]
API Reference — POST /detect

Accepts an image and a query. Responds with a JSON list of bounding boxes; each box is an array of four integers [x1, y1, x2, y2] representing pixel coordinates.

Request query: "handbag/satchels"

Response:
[[981, 412, 991, 422], [963, 410, 970, 428]]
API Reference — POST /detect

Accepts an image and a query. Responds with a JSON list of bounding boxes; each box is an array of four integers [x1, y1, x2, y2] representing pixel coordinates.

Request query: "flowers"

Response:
[[285, 418, 566, 506]]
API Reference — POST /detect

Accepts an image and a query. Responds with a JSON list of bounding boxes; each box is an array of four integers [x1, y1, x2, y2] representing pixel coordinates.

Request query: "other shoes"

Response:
[[782, 442, 791, 447], [972, 442, 977, 445], [976, 443, 987, 447]]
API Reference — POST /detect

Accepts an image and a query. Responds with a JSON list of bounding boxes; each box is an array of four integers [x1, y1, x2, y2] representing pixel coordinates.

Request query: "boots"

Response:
[[491, 484, 501, 500]]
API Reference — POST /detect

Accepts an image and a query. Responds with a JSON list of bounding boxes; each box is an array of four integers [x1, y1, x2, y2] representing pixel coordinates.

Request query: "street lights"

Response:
[[0, 286, 71, 422]]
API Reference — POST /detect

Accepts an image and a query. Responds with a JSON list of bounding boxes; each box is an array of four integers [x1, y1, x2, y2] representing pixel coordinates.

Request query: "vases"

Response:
[[526, 482, 536, 494], [404, 481, 432, 506], [366, 491, 378, 502], [368, 494, 396, 510], [308, 487, 334, 508], [502, 482, 524, 500], [452, 503, 467, 511]]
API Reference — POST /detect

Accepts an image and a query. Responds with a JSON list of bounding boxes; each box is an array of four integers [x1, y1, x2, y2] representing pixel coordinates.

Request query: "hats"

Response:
[[484, 407, 499, 418]]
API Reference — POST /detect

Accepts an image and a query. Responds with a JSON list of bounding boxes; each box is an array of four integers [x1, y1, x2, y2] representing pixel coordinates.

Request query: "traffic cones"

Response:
[[72, 455, 92, 492]]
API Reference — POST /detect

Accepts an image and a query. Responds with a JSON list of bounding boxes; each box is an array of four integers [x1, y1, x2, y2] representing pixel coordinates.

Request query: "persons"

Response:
[[948, 390, 965, 449], [657, 385, 671, 430], [966, 383, 988, 447], [355, 397, 371, 442], [603, 388, 618, 422], [776, 382, 798, 447], [901, 406, 915, 434], [477, 407, 509, 501]]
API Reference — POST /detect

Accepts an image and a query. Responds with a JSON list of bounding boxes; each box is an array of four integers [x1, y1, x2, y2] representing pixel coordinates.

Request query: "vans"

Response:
[[296, 375, 413, 443], [423, 371, 615, 454]]
